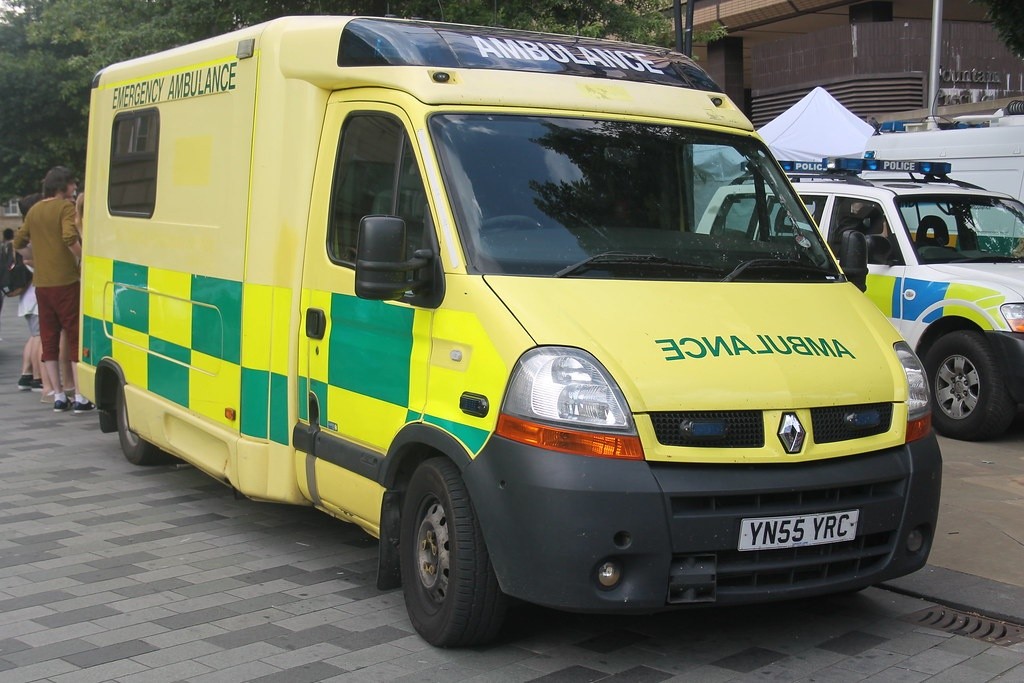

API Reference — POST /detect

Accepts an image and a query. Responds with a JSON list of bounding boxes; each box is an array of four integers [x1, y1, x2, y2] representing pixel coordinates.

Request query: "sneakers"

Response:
[[18, 375, 44, 395]]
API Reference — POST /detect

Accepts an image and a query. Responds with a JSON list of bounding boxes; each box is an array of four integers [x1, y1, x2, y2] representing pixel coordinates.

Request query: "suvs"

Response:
[[697, 157, 1023, 442]]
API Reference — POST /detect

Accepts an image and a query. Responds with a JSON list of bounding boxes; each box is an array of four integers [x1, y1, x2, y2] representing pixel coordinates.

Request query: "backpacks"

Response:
[[0, 243, 33, 299]]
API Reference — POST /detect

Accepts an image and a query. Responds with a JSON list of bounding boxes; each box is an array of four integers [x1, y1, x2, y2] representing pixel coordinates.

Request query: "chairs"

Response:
[[774, 206, 949, 264], [332, 154, 631, 282]]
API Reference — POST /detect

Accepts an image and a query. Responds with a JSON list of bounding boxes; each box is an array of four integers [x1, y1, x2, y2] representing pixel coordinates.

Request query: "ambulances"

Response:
[[70, 16, 945, 648]]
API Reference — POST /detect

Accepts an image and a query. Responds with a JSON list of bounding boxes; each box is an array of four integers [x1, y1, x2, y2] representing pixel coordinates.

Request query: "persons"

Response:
[[75, 193, 84, 239], [18, 193, 44, 392], [13, 166, 97, 413], [3, 228, 15, 247]]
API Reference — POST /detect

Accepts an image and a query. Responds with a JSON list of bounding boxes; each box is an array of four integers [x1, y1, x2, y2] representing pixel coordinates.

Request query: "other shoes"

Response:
[[64, 389, 77, 401], [41, 393, 55, 404], [55, 398, 71, 412], [73, 400, 96, 413]]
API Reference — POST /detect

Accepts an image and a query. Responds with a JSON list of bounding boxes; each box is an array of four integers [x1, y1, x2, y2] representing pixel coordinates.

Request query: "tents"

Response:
[[693, 87, 875, 227]]
